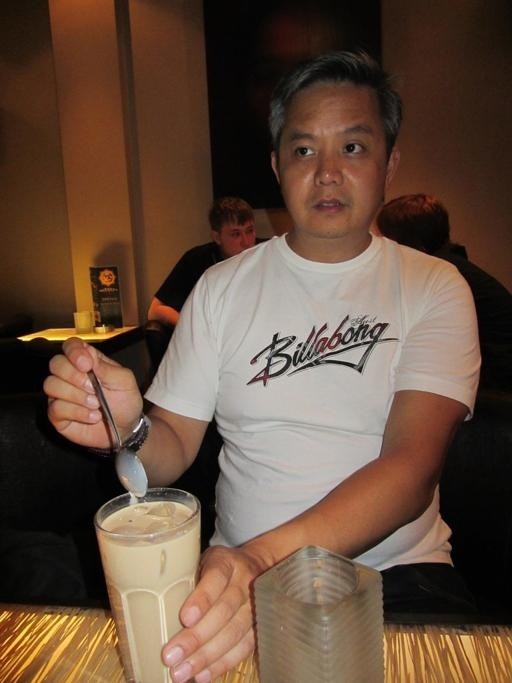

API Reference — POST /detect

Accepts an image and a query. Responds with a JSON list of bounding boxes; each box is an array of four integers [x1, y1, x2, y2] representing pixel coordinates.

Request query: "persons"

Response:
[[42, 47, 488, 682], [146, 194, 274, 332], [370, 190, 511, 396]]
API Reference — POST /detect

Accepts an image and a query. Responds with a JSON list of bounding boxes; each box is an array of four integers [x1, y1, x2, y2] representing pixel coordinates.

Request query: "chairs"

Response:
[[0, 312, 33, 336]]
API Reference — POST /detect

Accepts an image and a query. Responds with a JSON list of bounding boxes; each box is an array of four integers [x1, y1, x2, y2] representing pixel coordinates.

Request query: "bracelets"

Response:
[[89, 411, 152, 458]]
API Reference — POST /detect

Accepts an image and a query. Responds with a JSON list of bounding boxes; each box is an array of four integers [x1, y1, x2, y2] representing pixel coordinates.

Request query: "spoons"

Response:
[[87, 369, 148, 498]]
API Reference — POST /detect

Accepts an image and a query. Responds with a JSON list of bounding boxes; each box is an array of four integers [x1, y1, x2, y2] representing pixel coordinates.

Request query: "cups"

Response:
[[73, 311, 92, 334], [94, 487, 201, 683], [254, 544, 385, 683]]
